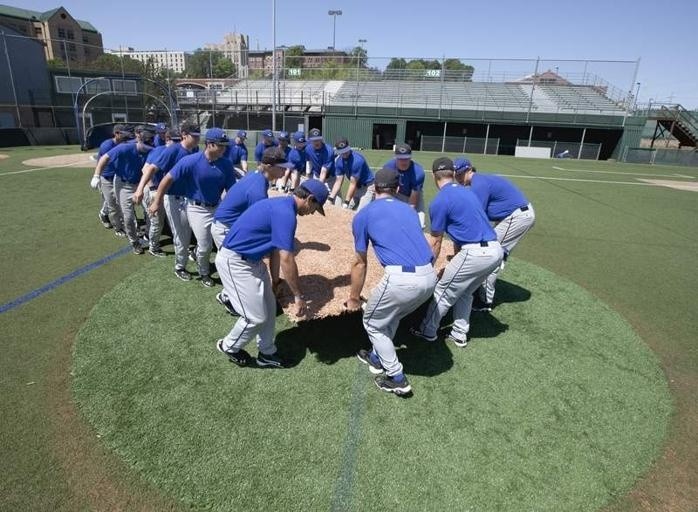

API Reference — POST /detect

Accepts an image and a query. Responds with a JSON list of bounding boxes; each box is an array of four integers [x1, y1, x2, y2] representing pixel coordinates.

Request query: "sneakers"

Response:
[[354, 300, 493, 397], [98, 211, 290, 369]]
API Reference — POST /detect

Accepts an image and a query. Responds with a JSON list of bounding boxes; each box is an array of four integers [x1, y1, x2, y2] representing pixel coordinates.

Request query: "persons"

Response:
[[349, 167, 437, 397], [217, 178, 330, 367], [92, 119, 375, 315], [407, 156, 507, 349], [383, 145, 426, 229], [450, 156, 538, 313]]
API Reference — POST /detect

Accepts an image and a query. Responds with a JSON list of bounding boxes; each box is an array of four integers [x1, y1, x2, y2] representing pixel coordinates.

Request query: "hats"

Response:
[[113, 118, 306, 168], [299, 128, 470, 216]]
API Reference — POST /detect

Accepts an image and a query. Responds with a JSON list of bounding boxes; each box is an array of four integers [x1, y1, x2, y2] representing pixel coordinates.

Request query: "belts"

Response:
[[480, 241, 488, 247], [520, 205, 528, 212], [402, 257, 436, 273]]
